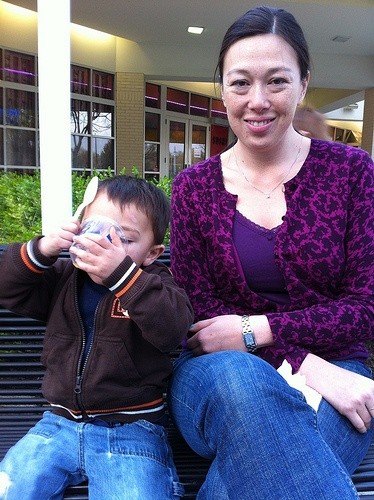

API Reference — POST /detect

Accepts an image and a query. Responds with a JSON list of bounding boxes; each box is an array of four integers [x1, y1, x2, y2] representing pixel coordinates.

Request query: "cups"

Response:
[[71, 217, 128, 268]]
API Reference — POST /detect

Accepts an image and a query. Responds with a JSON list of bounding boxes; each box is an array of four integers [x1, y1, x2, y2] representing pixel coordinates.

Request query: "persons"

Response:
[[0, 175, 194, 500], [170, 7, 374, 500]]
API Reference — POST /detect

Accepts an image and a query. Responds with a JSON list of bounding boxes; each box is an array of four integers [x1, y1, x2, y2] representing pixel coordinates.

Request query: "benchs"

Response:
[[0, 244, 374, 500]]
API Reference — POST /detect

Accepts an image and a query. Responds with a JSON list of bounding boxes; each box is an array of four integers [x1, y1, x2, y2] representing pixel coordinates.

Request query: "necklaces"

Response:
[[239, 135, 302, 200]]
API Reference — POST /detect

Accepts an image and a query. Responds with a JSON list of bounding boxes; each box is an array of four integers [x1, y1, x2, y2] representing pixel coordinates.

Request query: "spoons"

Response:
[[73, 176, 99, 219]]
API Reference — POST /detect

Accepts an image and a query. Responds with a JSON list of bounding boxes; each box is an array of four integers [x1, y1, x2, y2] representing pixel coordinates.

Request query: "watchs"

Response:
[[242, 315, 259, 354]]
